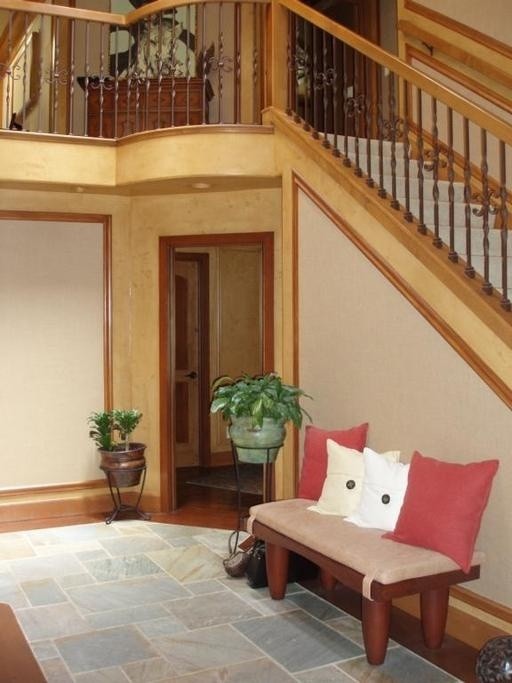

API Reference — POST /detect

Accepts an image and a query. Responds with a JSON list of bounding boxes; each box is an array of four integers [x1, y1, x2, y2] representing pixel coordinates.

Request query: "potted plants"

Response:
[[86, 406, 147, 490], [207, 368, 315, 464]]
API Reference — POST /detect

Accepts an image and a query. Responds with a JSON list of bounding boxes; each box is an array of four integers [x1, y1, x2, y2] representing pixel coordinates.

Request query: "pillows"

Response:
[[296, 422, 370, 501], [382, 449, 499, 576], [306, 437, 400, 519], [344, 446, 410, 534]]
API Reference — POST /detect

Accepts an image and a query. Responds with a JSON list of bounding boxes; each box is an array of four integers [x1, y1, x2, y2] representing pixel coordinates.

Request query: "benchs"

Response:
[[247, 494, 484, 667]]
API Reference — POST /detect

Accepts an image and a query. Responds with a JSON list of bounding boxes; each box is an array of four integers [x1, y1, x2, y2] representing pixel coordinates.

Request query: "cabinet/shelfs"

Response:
[[75, 74, 216, 138]]
